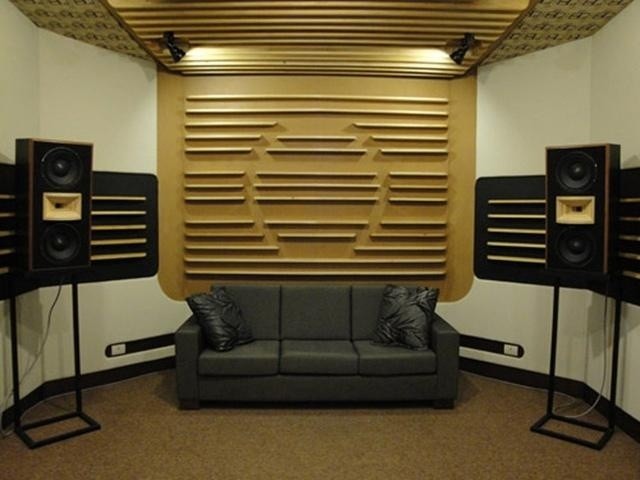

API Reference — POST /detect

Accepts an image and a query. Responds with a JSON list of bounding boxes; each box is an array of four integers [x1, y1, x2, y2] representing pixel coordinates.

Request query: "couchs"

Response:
[[171, 282, 461, 412]]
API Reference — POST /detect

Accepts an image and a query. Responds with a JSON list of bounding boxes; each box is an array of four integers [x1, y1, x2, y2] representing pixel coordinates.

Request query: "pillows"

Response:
[[368, 283, 440, 351], [184, 286, 255, 351]]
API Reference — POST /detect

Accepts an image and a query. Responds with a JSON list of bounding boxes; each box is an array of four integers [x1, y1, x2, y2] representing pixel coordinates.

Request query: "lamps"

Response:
[[156, 30, 187, 64], [449, 31, 481, 68]]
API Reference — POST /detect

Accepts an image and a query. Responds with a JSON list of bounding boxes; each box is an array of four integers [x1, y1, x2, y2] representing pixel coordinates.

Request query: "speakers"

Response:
[[14, 136, 93, 274], [545, 144, 621, 277]]
